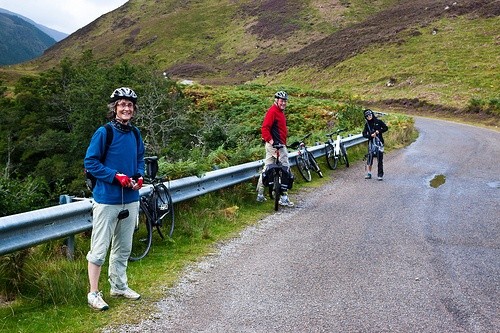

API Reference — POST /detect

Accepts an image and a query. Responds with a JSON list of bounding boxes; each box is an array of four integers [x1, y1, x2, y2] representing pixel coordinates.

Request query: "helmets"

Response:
[[108, 87, 138, 104], [364, 109, 373, 116], [274, 91, 288, 101]]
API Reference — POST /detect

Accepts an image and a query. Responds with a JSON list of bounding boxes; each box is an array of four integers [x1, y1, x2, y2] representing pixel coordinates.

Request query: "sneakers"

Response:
[[87, 290, 109, 312], [110, 287, 141, 300], [256, 195, 268, 201], [278, 197, 294, 208]]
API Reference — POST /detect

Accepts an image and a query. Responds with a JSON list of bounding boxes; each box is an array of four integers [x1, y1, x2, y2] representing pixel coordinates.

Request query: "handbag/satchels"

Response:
[[279, 165, 295, 193], [261, 163, 278, 187]]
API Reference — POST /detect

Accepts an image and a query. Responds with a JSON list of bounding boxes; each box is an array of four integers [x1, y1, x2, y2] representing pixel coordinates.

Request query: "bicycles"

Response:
[[324, 128, 350, 169], [128, 155, 175, 262], [288, 133, 324, 183], [269, 144, 286, 210]]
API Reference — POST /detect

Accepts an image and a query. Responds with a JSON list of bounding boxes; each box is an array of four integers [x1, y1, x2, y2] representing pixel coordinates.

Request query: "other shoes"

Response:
[[365, 173, 371, 179], [377, 176, 383, 180]]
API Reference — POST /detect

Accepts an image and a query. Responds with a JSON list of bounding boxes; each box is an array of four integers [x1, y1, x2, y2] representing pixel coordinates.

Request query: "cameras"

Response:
[[127, 178, 138, 187]]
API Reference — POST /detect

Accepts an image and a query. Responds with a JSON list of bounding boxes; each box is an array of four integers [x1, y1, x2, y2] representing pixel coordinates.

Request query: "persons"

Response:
[[362, 109, 389, 181], [83, 88, 145, 311], [256, 91, 294, 207]]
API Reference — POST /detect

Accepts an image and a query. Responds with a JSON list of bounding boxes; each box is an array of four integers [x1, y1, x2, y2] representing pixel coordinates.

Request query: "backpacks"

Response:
[[366, 136, 385, 166], [84, 123, 140, 193]]
